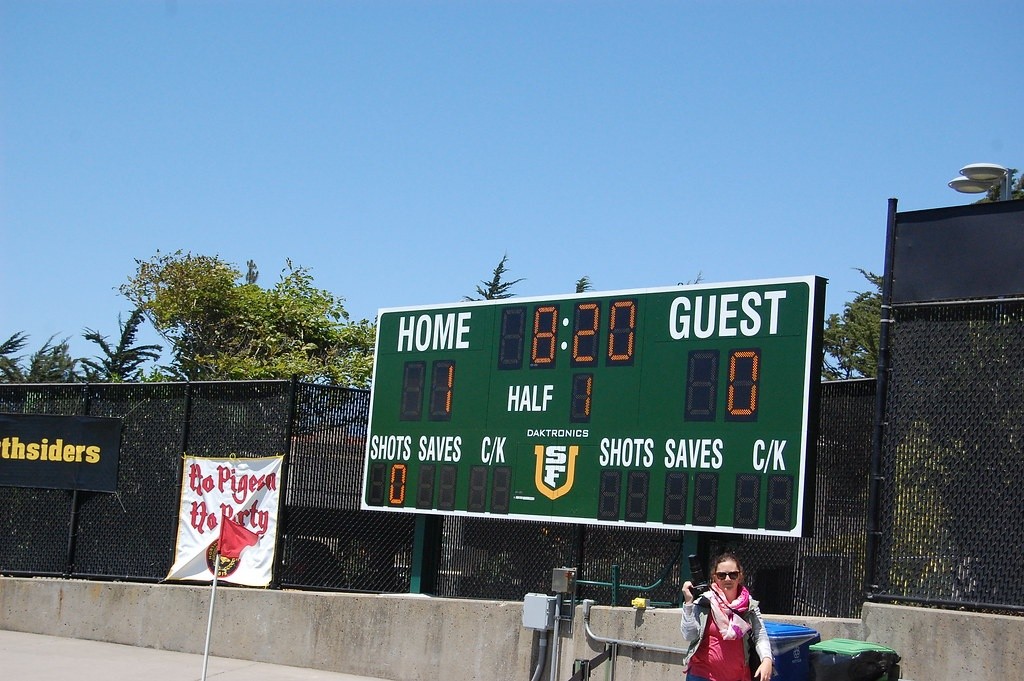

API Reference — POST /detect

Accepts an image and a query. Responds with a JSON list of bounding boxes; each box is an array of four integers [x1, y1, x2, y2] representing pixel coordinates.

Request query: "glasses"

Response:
[[714, 572, 740, 580]]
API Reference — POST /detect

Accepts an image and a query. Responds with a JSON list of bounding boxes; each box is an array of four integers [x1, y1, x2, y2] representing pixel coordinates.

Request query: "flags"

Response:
[[221, 508, 258, 558]]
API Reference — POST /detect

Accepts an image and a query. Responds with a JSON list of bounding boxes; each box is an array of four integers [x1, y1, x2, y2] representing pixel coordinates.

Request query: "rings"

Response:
[[769, 677, 770, 679]]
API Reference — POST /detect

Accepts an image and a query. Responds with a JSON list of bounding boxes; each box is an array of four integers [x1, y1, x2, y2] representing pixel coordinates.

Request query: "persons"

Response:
[[682, 554, 773, 681]]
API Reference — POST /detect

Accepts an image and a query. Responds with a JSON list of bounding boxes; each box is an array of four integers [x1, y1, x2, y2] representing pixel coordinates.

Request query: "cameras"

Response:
[[687, 554, 711, 593]]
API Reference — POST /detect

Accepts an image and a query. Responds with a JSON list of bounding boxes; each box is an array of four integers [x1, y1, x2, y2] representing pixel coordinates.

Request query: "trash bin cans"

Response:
[[750, 622, 820, 681], [809, 637, 902, 681]]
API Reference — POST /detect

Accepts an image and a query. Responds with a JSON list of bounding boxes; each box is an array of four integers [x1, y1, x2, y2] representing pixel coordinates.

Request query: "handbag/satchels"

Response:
[[747, 628, 778, 681]]
